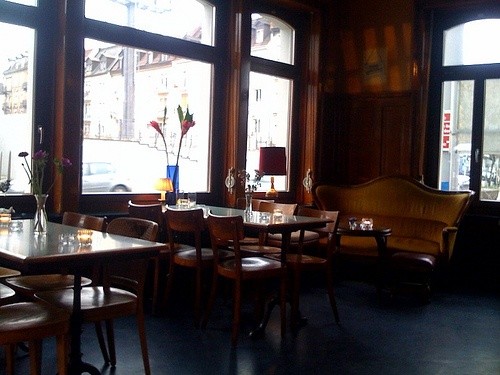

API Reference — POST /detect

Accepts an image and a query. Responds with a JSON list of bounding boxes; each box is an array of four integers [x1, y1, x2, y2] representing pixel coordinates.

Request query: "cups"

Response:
[[272, 208, 284, 222], [188, 192, 196, 208], [176, 188, 184, 206], [361, 217, 373, 230], [0, 208, 11, 226], [77, 228, 93, 247]]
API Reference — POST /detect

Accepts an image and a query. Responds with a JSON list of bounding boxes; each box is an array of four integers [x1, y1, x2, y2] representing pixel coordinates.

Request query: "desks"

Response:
[[163, 203, 334, 340], [0, 218, 167, 375], [335, 226, 391, 284]]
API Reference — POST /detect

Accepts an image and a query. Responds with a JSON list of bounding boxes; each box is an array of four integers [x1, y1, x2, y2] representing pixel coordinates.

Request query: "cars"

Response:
[[9, 155, 135, 193]]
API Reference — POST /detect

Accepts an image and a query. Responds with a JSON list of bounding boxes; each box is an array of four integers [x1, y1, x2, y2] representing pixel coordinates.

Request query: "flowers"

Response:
[[238, 171, 264, 194], [18, 151, 72, 195], [149, 108, 196, 166]]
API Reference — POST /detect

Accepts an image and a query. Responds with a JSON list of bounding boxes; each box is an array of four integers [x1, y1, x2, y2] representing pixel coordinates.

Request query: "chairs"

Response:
[[231, 203, 297, 255], [262, 206, 340, 326], [239, 198, 275, 244], [165, 207, 235, 328], [200, 209, 287, 346], [6, 212, 109, 353], [31, 218, 157, 375], [128, 200, 193, 305], [0, 303, 70, 374]]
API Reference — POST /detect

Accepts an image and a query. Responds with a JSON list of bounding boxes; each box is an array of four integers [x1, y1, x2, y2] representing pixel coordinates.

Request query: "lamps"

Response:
[[260, 147, 286, 199]]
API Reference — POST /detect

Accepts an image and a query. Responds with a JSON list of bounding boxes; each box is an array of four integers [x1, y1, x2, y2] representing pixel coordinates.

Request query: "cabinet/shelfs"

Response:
[[325, 91, 416, 186]]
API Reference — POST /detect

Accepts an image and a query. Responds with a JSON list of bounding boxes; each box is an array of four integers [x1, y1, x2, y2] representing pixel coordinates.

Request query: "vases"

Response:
[[35, 195, 48, 235], [245, 194, 252, 214], [166, 166, 178, 204]]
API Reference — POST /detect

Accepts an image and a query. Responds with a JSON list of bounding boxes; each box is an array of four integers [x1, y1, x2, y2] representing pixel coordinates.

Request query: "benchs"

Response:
[[312, 173, 467, 285]]
